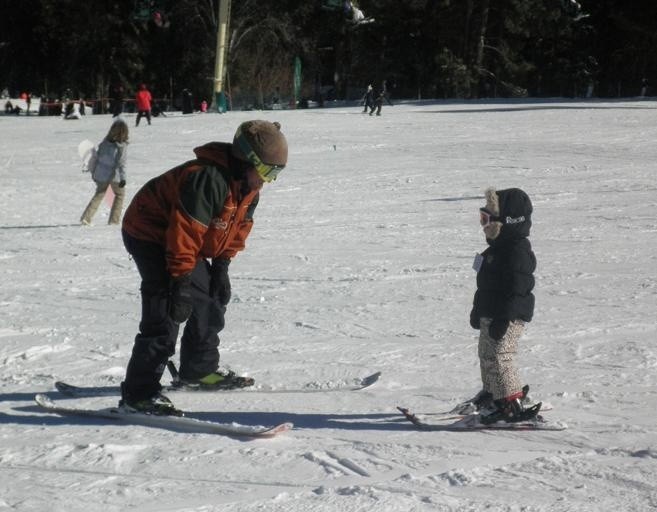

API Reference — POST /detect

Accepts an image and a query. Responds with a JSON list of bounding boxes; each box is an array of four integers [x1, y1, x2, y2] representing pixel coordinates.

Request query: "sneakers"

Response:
[[478, 395, 526, 424], [130, 395, 179, 417], [197, 366, 239, 387]]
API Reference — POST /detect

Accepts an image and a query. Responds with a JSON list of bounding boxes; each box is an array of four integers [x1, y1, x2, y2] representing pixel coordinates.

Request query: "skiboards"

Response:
[[395, 405, 569, 431], [37, 371, 384, 439]]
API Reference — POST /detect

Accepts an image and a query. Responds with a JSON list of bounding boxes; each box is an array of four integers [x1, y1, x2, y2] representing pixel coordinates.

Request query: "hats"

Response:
[[235, 119, 289, 166]]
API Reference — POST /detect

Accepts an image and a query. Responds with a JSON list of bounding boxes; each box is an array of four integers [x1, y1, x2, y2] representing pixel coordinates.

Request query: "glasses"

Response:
[[479, 208, 503, 228], [236, 130, 283, 185]]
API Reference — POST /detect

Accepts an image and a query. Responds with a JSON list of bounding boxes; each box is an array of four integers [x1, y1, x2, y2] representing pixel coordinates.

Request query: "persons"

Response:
[[79, 118, 132, 226], [116, 119, 290, 417], [459, 187, 537, 424]]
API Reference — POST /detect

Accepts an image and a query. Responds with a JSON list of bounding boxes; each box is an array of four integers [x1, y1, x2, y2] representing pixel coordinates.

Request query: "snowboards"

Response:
[[79, 139, 99, 175]]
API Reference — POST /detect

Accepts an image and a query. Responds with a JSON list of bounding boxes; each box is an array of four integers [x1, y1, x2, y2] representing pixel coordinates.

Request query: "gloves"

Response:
[[469, 305, 485, 329], [488, 314, 511, 340], [204, 258, 231, 306], [164, 275, 194, 323]]
